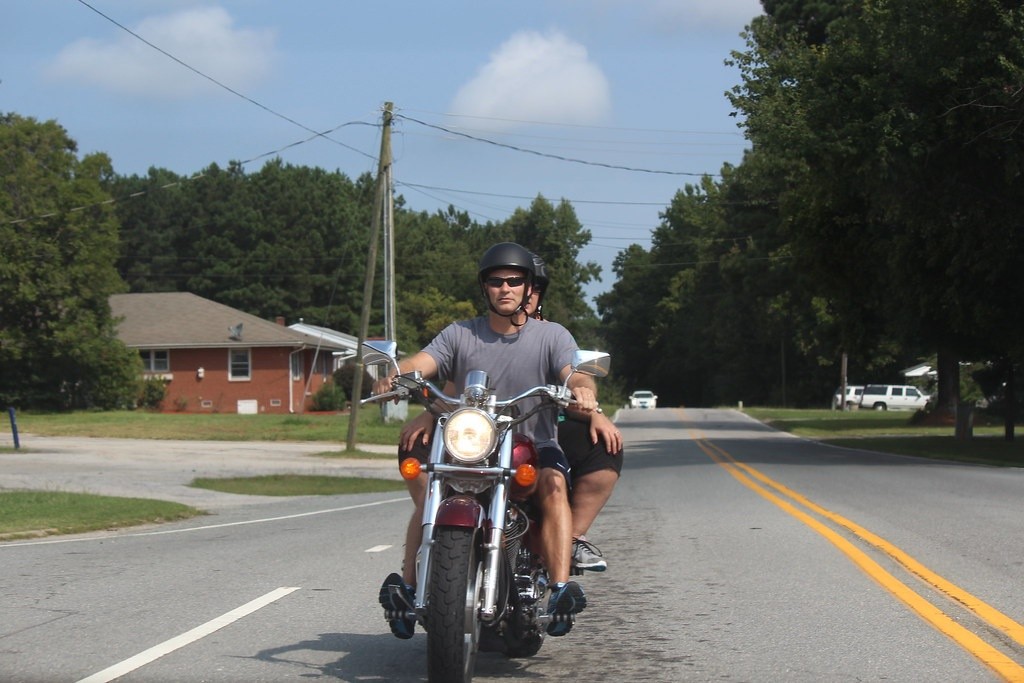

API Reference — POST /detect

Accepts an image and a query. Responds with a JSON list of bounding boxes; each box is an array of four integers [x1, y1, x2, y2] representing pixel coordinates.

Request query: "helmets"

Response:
[[477, 241, 535, 282], [529, 251, 549, 291]]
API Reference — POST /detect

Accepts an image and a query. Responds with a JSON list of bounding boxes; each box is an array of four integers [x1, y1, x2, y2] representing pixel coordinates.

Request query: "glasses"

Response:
[[532, 283, 543, 294], [486, 276, 526, 287]]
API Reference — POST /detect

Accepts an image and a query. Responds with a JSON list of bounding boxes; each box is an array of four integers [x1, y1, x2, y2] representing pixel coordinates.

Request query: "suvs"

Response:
[[836, 386, 863, 410], [861, 384, 931, 412]]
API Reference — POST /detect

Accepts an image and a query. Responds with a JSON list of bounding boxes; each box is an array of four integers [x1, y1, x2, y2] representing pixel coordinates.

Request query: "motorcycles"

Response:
[[361, 339, 612, 683]]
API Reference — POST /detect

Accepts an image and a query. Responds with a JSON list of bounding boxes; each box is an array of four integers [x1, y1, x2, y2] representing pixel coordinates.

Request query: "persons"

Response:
[[397, 249, 623, 571], [373, 242, 599, 640]]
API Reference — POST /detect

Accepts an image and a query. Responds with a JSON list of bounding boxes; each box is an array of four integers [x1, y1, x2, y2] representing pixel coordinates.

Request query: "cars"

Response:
[[629, 391, 657, 409]]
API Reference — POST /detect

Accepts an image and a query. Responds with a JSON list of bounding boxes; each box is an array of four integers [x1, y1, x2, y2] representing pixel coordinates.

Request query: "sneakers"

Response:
[[379, 572, 416, 639], [572, 535, 608, 572], [544, 581, 588, 637]]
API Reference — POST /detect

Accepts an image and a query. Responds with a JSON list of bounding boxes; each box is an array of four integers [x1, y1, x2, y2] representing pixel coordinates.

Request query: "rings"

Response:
[[588, 394, 593, 398]]
